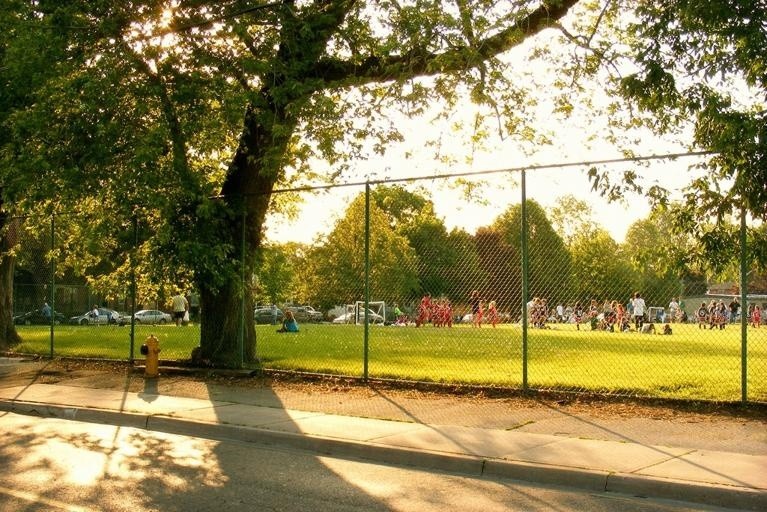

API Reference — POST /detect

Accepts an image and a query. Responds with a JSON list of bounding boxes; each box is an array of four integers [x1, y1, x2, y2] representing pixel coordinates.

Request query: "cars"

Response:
[[13, 308, 65, 326], [333, 313, 353, 323], [123, 310, 172, 325], [68, 309, 122, 326]]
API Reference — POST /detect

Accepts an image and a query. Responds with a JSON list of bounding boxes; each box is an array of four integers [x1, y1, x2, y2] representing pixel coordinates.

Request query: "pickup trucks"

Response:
[[301, 306, 324, 322]]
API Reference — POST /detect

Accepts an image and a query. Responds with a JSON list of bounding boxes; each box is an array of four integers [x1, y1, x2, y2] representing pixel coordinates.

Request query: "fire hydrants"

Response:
[[140, 334, 161, 378]]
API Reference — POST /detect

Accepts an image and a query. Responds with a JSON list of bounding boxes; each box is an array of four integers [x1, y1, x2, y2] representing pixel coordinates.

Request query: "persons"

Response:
[[107, 308, 111, 325], [170, 292, 189, 327], [468, 290, 483, 327], [519, 289, 688, 335], [697, 293, 759, 330], [270, 304, 277, 325], [489, 299, 496, 327], [275, 311, 298, 332], [42, 302, 52, 324], [91, 305, 99, 325], [476, 298, 486, 327], [394, 292, 454, 328]]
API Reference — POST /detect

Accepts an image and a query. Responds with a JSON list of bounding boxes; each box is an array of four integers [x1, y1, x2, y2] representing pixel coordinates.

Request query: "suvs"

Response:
[[253, 308, 283, 325], [284, 307, 309, 322]]
